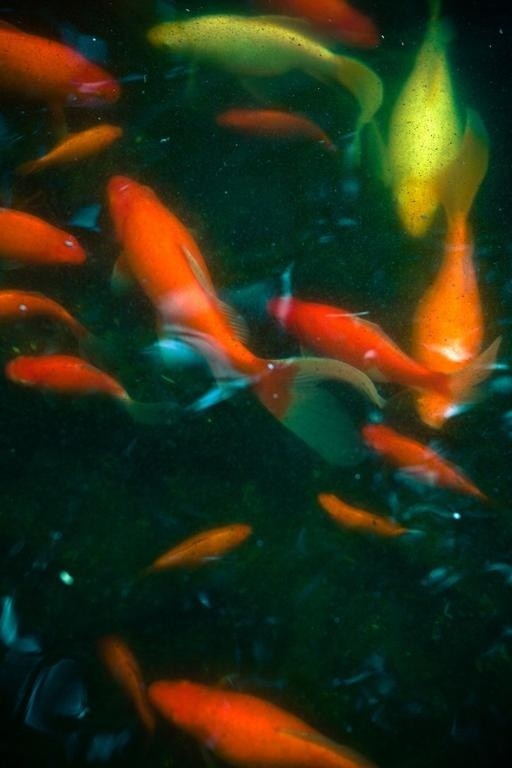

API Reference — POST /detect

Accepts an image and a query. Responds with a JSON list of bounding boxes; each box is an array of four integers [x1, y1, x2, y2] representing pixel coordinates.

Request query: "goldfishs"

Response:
[[0, 0, 512, 504]]
